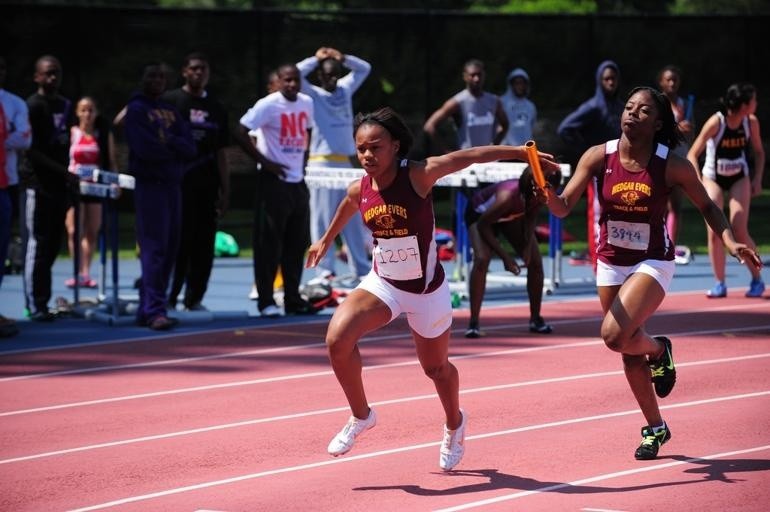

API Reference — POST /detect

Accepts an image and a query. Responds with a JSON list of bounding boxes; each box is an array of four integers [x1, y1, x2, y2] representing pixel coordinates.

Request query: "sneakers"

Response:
[[646, 336, 675, 397], [261, 304, 282, 317], [746, 280, 764, 297], [149, 314, 172, 329], [529, 318, 552, 333], [635, 420, 671, 459], [706, 280, 726, 297], [285, 301, 314, 315], [328, 408, 376, 457], [466, 326, 479, 337], [439, 409, 465, 471]]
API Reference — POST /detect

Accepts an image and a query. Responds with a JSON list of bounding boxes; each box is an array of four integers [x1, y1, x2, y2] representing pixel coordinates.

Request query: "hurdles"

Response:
[[450, 168, 564, 295], [456, 162, 571, 288], [75, 169, 249, 328], [305, 174, 479, 300]]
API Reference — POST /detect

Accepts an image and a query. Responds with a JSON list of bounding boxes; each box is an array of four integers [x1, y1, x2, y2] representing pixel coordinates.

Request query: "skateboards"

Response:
[[305, 165, 470, 293]]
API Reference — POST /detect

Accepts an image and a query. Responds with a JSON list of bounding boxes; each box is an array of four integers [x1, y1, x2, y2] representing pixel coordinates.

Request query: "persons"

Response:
[[1, 48, 235, 332], [239, 47, 378, 315], [301, 109, 560, 468], [535, 86, 763, 461], [420, 57, 693, 294]]
